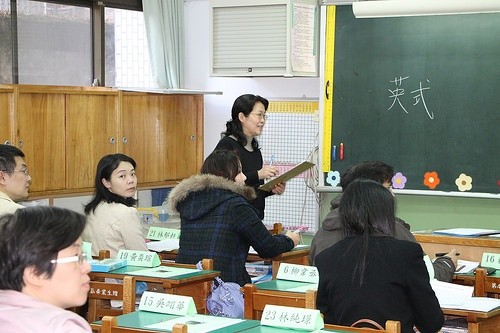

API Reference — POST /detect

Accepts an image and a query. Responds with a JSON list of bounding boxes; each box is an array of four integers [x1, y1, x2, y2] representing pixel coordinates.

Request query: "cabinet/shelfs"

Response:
[[0, 82, 206, 205]]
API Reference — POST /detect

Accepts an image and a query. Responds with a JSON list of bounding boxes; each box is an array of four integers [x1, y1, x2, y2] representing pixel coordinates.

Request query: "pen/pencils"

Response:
[[266, 155, 273, 181], [293, 228, 302, 234], [435, 253, 460, 257]]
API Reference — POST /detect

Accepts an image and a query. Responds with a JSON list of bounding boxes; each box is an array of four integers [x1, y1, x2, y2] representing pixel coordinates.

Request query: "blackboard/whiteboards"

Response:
[[316, 4, 500, 198]]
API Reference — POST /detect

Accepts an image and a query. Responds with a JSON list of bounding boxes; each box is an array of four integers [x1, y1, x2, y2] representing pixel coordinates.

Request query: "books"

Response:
[[441, 295, 500, 312], [433, 257, 479, 275], [421, 254, 474, 304]]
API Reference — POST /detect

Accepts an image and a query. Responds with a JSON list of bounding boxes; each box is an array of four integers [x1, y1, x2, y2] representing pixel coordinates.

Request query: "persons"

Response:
[[314, 178, 445, 333], [0, 145, 33, 216], [164, 151, 301, 287], [0, 205, 92, 333], [212, 95, 286, 221], [83, 154, 149, 253], [308, 160, 458, 281]]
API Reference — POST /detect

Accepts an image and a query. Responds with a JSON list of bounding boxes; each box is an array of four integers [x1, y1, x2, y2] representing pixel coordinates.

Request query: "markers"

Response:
[[333, 145, 336, 161], [340, 143, 344, 160]]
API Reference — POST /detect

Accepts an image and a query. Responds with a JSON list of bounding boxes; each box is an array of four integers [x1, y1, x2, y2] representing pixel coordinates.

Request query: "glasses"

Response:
[[50, 251, 90, 267], [250, 112, 268, 119], [2, 169, 28, 175]]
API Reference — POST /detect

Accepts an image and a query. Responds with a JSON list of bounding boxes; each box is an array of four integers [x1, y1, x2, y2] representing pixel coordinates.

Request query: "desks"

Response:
[[65, 227, 500, 333]]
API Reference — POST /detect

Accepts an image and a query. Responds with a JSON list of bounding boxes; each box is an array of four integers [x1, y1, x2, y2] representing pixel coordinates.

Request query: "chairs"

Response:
[[158, 251, 218, 272], [241, 280, 318, 321], [69, 275, 137, 325]]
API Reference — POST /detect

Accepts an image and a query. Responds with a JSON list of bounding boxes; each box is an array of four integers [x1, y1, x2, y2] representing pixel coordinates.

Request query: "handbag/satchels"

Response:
[[196, 259, 245, 318]]
